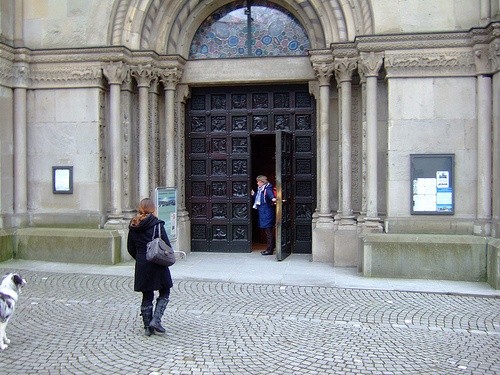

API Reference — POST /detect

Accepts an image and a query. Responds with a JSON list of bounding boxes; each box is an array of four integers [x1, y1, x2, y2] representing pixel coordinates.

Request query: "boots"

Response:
[[140, 304, 155, 336], [149, 297, 170, 333]]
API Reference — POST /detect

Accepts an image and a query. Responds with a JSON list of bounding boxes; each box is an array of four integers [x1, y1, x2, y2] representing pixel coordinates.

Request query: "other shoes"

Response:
[[261, 251, 273, 255]]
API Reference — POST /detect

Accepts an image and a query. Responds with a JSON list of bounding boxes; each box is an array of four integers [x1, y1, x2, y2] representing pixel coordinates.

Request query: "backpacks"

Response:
[[264, 183, 276, 206]]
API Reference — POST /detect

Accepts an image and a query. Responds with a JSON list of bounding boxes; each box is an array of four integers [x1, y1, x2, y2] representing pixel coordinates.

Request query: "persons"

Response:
[[251, 175, 277, 255], [127, 198, 176, 336], [264, 160, 277, 205]]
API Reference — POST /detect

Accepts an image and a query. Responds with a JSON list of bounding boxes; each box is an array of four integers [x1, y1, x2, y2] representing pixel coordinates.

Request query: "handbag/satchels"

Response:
[[146, 222, 175, 266]]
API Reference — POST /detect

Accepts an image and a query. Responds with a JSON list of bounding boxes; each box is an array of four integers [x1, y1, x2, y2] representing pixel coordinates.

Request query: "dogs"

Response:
[[0, 270, 27, 351]]
